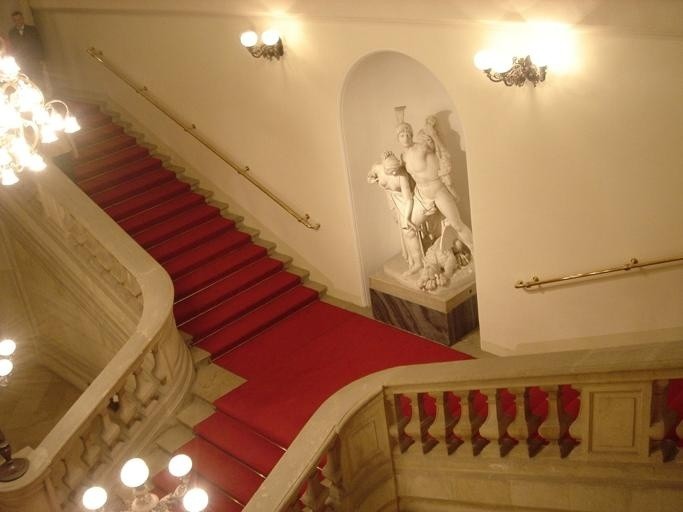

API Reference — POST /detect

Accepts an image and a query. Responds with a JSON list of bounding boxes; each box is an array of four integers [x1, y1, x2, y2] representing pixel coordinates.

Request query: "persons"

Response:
[[370, 123, 470, 289], [8, 12, 47, 99]]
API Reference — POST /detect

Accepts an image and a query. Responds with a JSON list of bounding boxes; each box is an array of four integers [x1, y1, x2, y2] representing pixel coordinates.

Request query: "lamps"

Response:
[[240, 27, 283, 62], [0, 36, 79, 190], [0, 337, 31, 480], [79, 452, 209, 512], [468, 41, 549, 87]]
